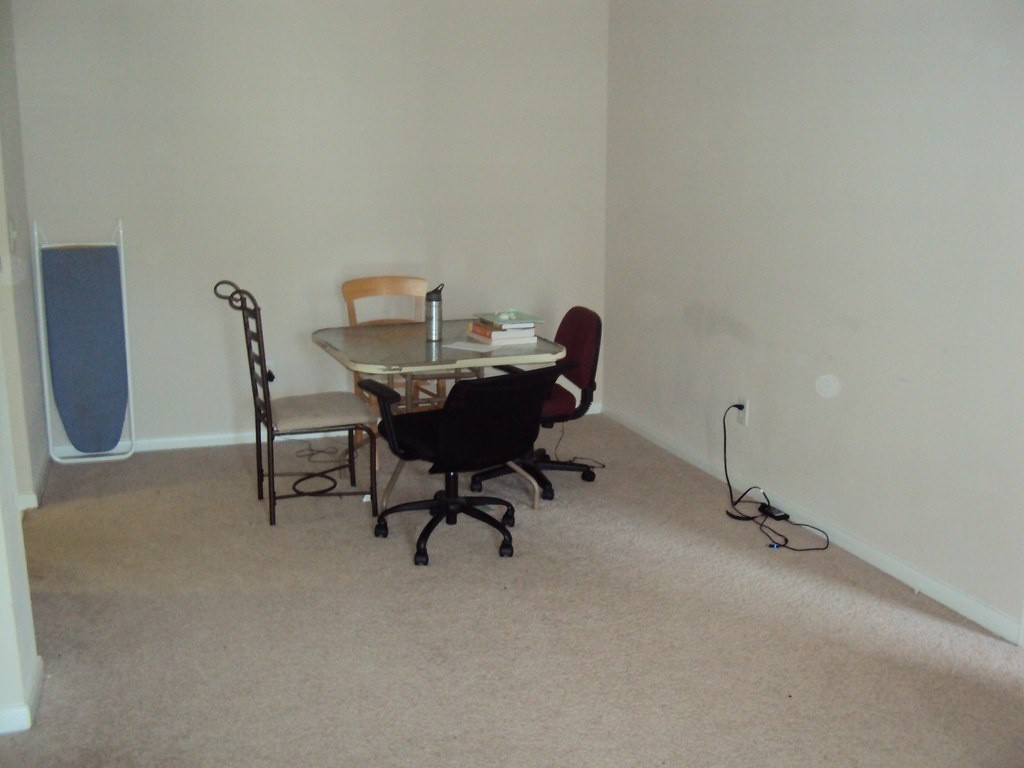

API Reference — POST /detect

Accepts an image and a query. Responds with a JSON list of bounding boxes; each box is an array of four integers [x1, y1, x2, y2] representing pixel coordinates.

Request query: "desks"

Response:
[[311, 319, 566, 514]]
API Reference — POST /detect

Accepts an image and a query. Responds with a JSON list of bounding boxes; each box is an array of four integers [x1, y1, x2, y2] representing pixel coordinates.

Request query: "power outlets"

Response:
[[736, 398, 749, 426]]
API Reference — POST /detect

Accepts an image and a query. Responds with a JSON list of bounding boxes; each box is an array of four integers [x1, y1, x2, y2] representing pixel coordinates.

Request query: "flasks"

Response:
[[425, 283, 445, 343]]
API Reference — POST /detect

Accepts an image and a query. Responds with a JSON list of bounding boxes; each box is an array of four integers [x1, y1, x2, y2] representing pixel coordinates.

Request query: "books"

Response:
[[466, 311, 544, 346]]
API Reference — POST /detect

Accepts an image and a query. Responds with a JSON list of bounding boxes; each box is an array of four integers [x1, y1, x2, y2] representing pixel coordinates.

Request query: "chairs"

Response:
[[341, 276, 446, 441], [357, 365, 555, 566], [213, 280, 378, 526], [470, 306, 602, 501]]
